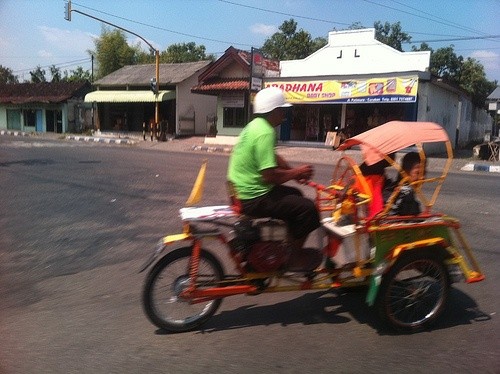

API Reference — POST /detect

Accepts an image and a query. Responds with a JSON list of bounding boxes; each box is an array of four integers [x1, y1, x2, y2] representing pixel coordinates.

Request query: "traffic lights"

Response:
[[62, 1, 159, 143]]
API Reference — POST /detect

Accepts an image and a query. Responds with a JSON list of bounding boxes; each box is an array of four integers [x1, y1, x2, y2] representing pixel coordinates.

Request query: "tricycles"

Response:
[[138, 87, 487, 332]]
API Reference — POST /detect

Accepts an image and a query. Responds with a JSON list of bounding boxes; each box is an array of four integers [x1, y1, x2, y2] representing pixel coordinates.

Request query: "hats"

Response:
[[253, 87, 293, 114]]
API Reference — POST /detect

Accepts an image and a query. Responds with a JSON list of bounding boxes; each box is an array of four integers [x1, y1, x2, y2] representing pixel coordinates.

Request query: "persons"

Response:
[[226, 88, 321, 273], [359, 152, 424, 223]]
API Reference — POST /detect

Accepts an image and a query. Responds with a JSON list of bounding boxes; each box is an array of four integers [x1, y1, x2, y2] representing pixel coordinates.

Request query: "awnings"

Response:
[[84, 89, 176, 102]]
